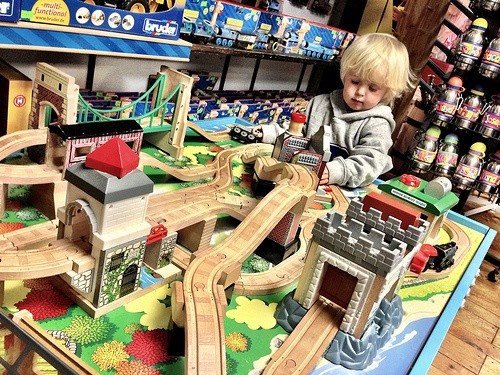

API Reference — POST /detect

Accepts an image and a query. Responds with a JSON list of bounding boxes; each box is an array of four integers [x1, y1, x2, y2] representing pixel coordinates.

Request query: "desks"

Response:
[[0, 117, 497, 375]]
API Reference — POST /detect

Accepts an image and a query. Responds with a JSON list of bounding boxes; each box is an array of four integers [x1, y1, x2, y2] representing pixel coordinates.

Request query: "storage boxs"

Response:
[[0, 0, 360, 94]]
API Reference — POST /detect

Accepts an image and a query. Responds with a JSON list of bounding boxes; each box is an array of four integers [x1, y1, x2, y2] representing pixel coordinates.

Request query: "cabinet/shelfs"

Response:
[[0, 0, 359, 205], [309, 0, 500, 205]]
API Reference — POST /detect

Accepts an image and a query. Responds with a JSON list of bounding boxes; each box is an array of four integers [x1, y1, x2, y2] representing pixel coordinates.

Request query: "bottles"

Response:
[[434, 75, 466, 123], [479, 29, 500, 80], [453, 90, 485, 131], [410, 126, 442, 173], [482, 0, 500, 11], [452, 141, 487, 191], [434, 133, 459, 177], [474, 94, 500, 142], [476, 150, 500, 196], [454, 18, 487, 72]]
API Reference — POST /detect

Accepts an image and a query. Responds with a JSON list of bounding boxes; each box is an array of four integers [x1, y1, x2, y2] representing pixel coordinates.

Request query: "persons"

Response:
[[253, 33, 417, 189]]
